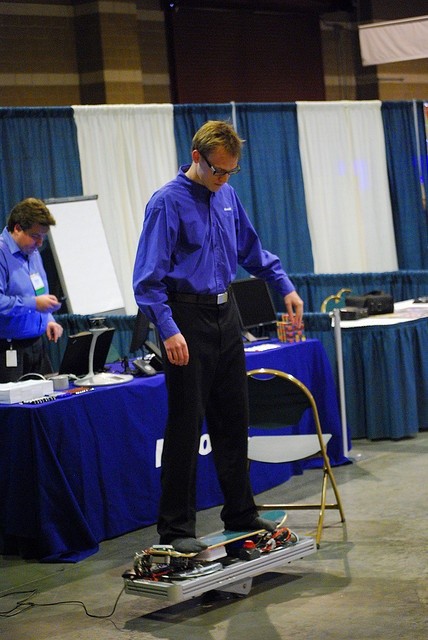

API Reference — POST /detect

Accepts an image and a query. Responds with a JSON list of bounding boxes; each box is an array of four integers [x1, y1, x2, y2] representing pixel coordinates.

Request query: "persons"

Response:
[[131, 120, 308, 554], [0, 195, 64, 382]]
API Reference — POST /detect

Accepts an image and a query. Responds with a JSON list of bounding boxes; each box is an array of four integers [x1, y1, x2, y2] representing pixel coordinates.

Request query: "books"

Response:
[[0, 379, 55, 403]]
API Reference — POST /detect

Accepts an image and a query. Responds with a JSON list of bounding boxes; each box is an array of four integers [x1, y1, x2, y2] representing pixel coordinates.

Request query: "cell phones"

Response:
[[131, 360, 157, 376]]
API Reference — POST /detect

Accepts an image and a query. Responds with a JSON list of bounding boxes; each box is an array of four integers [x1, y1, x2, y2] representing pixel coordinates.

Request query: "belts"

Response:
[[167, 290, 230, 305]]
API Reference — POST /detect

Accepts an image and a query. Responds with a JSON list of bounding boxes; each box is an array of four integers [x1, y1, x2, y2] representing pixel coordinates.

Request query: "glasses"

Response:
[[201, 154, 242, 176]]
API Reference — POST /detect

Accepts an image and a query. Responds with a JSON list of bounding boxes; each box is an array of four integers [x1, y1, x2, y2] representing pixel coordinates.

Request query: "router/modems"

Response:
[[341, 306, 368, 320]]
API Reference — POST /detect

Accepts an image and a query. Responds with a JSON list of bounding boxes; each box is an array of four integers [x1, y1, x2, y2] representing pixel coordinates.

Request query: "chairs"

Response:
[[247, 369, 344, 549]]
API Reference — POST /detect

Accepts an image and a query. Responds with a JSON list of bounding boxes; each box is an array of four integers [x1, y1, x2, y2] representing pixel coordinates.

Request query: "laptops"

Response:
[[42, 327, 115, 379]]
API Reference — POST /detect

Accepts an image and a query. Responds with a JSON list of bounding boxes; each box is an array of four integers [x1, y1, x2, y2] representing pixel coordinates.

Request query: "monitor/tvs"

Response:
[[130, 306, 164, 370], [229, 277, 279, 343]]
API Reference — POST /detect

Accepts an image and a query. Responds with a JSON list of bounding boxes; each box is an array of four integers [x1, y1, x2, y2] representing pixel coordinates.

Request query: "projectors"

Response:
[[346, 291, 394, 315]]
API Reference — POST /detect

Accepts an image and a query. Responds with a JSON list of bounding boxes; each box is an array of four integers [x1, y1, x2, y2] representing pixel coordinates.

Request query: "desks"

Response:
[[304, 294, 428, 441], [0, 336, 353, 561]]
